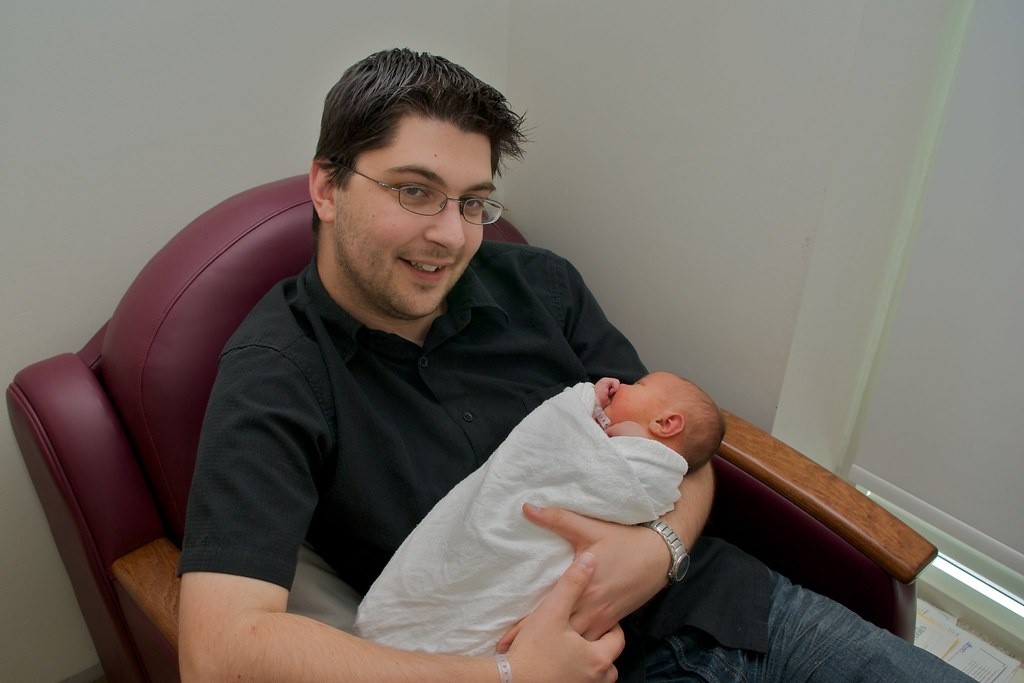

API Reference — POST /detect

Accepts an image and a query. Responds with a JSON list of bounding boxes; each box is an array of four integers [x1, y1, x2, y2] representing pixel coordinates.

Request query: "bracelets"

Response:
[[494, 654, 512, 683], [592, 393, 611, 429]]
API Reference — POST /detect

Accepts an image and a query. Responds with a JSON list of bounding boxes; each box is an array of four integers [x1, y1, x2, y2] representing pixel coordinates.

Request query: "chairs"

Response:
[[6, 172, 939, 683]]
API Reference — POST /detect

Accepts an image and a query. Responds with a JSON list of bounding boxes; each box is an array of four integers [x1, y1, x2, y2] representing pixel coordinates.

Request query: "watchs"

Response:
[[638, 520, 690, 583]]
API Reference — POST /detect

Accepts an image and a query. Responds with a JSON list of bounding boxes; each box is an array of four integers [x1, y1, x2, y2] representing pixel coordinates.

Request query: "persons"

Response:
[[353, 372, 725, 654], [178, 47, 986, 683]]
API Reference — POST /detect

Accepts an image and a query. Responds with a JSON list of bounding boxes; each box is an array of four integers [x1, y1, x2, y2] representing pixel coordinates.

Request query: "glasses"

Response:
[[331, 161, 508, 225]]
